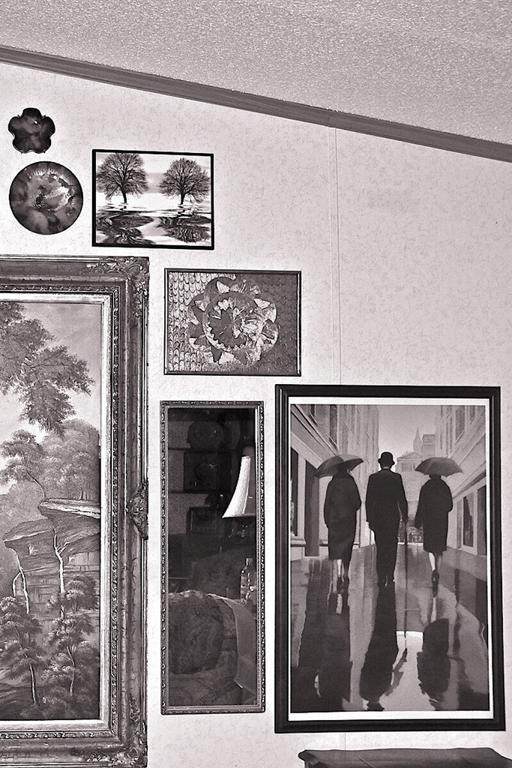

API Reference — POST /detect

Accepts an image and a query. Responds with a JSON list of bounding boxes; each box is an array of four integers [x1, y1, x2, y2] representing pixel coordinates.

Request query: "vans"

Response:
[[432, 569, 440, 584], [337, 574, 350, 585]]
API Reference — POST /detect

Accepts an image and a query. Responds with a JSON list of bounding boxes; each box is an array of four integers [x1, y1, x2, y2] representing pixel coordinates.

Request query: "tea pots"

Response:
[[158, 399, 268, 718]]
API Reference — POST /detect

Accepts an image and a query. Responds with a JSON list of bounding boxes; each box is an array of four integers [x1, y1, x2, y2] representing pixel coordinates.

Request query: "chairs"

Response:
[[378, 575, 394, 588]]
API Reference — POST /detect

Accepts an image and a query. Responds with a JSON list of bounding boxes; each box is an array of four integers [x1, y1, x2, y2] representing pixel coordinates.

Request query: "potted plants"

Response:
[[89, 148, 218, 251], [270, 382, 506, 734], [1, 249, 155, 768], [164, 266, 304, 378]]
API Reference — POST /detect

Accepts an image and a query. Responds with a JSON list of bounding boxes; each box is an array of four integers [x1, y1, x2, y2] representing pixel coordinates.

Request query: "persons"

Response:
[[366, 451, 408, 586], [358, 582, 399, 711], [324, 465, 360, 583], [418, 586, 452, 705], [416, 475, 453, 582], [320, 580, 351, 708]]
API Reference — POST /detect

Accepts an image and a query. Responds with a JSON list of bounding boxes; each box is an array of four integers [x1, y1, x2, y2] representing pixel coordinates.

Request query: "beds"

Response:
[[313, 453, 364, 479], [414, 457, 463, 477]]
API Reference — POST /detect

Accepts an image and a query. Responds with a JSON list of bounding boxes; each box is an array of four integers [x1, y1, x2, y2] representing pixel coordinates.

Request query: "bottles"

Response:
[[378, 452, 395, 465]]
